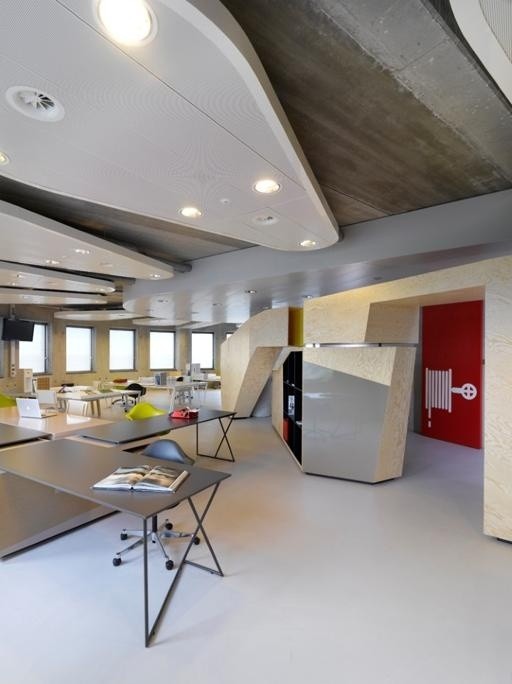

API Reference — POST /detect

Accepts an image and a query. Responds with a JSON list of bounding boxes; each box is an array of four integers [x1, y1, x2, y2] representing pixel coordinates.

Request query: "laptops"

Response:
[[15, 398, 57, 418]]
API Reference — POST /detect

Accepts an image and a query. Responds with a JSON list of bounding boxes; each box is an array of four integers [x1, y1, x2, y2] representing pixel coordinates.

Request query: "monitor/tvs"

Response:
[[0, 318, 35, 341]]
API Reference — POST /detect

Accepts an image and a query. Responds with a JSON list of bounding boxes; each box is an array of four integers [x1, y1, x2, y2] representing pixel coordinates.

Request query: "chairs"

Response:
[[112, 440, 200, 570], [36, 389, 57, 410]]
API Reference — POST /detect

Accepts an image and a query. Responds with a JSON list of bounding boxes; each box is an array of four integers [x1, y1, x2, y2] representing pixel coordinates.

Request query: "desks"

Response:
[[81, 409, 237, 462], [2, 438, 232, 648], [1, 421, 54, 447], [49, 372, 221, 417]]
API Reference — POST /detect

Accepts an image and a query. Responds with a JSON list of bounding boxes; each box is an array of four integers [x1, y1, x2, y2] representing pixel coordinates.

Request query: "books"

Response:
[[287, 394, 295, 416], [90, 463, 192, 494]]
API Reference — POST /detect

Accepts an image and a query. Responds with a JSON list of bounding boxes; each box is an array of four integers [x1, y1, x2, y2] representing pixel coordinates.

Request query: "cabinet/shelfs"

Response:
[[283, 351, 303, 465]]
